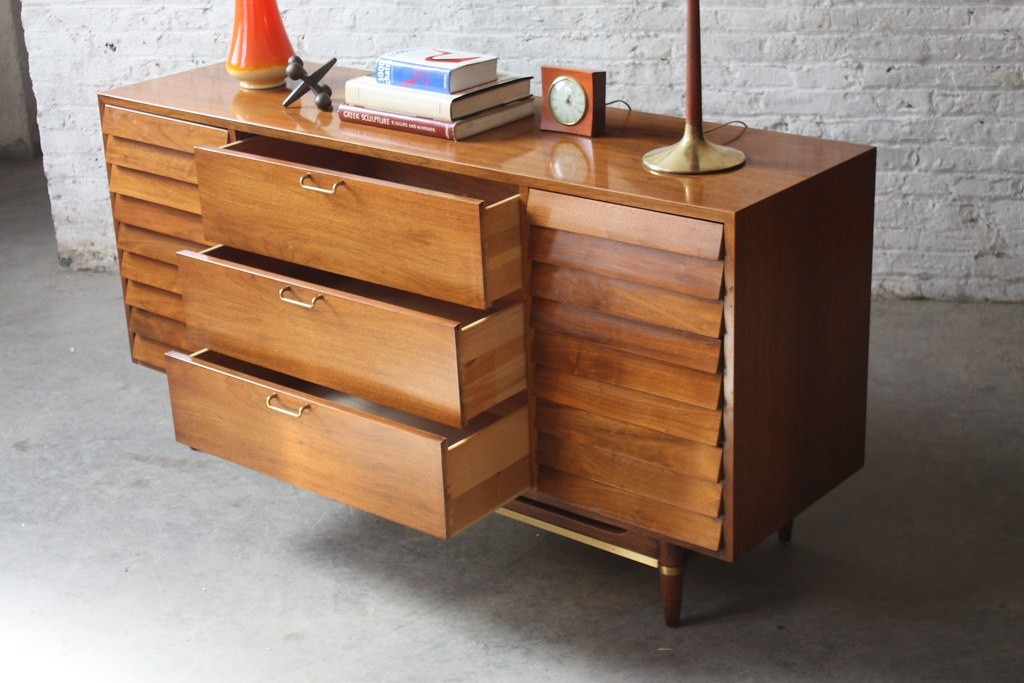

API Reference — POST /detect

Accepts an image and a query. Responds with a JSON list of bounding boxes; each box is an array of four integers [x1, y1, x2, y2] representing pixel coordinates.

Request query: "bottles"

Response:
[[226, 0, 295, 89]]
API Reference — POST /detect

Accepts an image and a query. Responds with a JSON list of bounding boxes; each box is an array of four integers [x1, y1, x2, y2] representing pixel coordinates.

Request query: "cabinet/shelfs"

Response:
[[97, 58, 877, 631]]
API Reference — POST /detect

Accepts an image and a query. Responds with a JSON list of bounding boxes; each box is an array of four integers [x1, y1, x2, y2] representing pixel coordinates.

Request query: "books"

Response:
[[344, 72, 533, 122], [337, 95, 536, 141], [376, 48, 500, 93]]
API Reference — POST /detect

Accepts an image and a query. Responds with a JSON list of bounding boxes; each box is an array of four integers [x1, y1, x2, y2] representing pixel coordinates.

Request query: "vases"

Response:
[[226, 0, 297, 91]]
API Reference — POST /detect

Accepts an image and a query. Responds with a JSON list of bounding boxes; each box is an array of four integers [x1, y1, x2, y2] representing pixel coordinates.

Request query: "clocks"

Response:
[[549, 139, 608, 189], [539, 63, 607, 137]]
